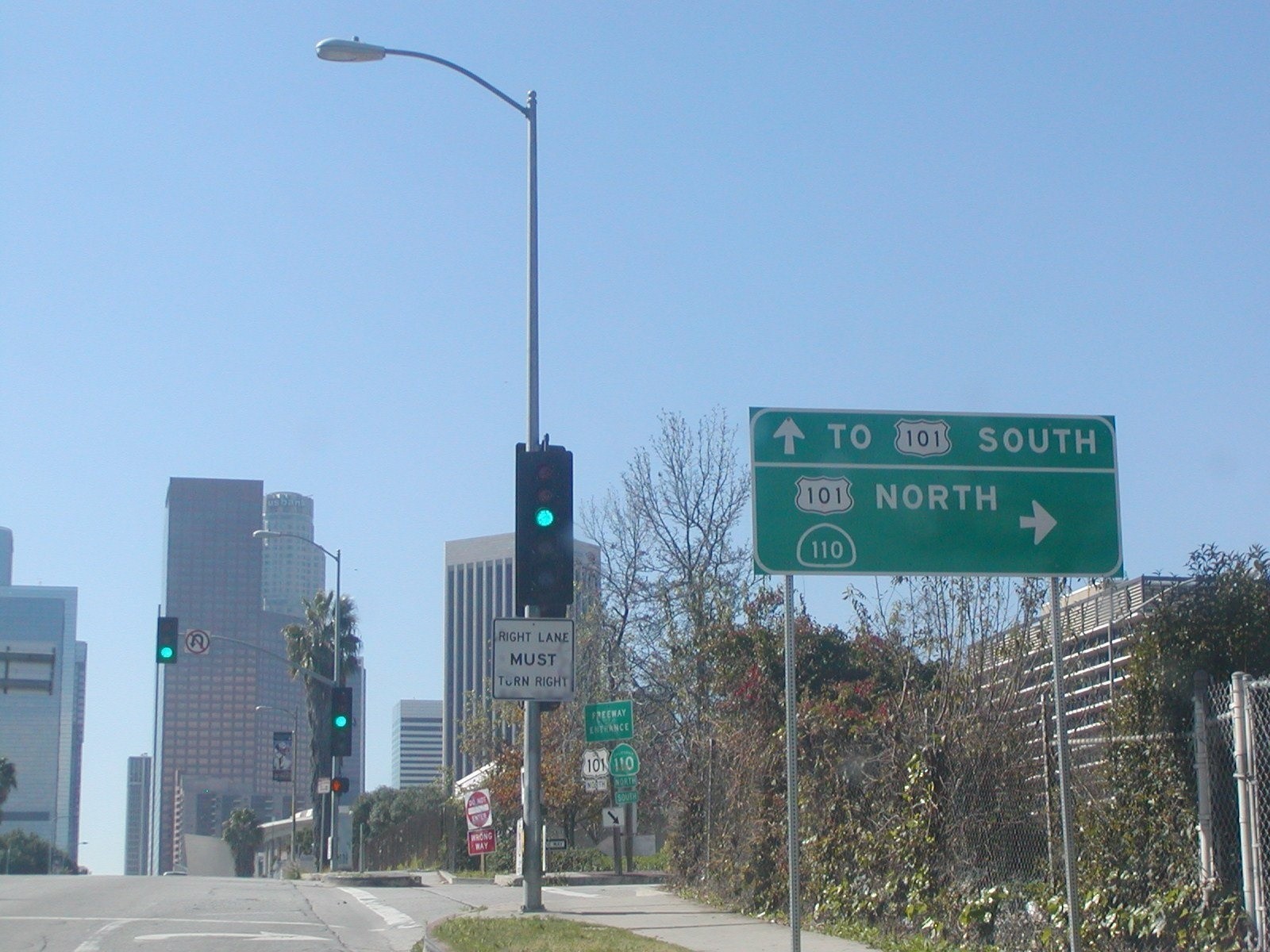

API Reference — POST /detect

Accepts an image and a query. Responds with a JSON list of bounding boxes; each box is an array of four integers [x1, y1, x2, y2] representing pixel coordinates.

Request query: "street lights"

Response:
[[256, 705, 298, 881], [253, 530, 342, 871], [320, 36, 550, 915]]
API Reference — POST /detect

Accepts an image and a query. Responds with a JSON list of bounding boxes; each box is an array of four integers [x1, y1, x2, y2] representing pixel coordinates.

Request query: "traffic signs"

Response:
[[747, 405, 1129, 583]]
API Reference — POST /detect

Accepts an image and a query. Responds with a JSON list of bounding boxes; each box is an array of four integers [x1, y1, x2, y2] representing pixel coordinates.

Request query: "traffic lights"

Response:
[[328, 777, 348, 795], [65, 839, 88, 875], [155, 617, 180, 665], [48, 812, 81, 872], [331, 685, 351, 758], [462, 787, 494, 830], [510, 444, 573, 609]]
